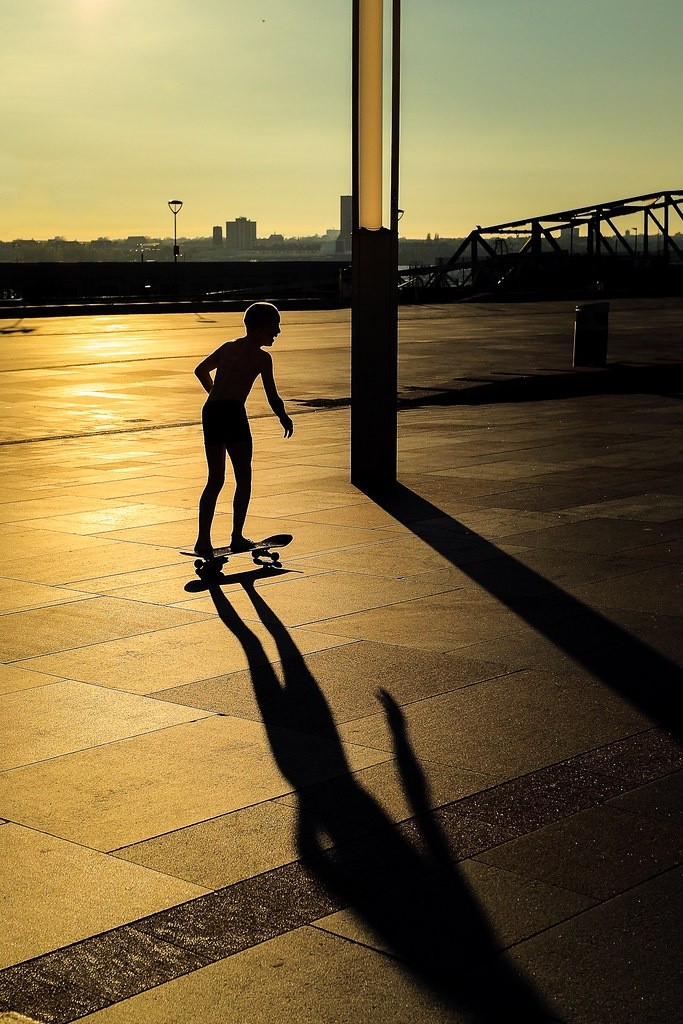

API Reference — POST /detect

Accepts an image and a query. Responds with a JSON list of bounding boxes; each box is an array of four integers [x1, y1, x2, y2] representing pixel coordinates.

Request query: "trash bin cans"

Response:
[[570, 300, 610, 372]]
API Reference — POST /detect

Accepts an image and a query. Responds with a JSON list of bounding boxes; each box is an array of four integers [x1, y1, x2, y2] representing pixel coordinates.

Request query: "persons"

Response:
[[193, 302, 293, 559]]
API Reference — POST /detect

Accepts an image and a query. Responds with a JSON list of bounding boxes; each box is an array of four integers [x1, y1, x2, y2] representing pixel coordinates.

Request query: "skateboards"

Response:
[[180, 533, 294, 568]]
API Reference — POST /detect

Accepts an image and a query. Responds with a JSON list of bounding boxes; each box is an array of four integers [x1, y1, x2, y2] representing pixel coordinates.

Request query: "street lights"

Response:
[[632, 228, 637, 250], [169, 201, 182, 262]]
